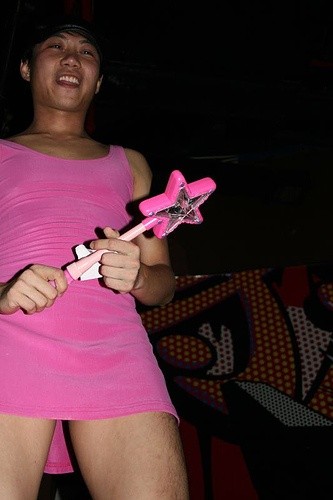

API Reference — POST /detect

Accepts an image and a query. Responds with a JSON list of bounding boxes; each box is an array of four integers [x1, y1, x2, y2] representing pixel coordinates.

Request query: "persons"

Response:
[[0, 15, 192, 500]]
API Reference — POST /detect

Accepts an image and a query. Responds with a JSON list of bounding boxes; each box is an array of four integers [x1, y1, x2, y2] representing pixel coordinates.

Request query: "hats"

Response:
[[29, 18, 104, 64]]
